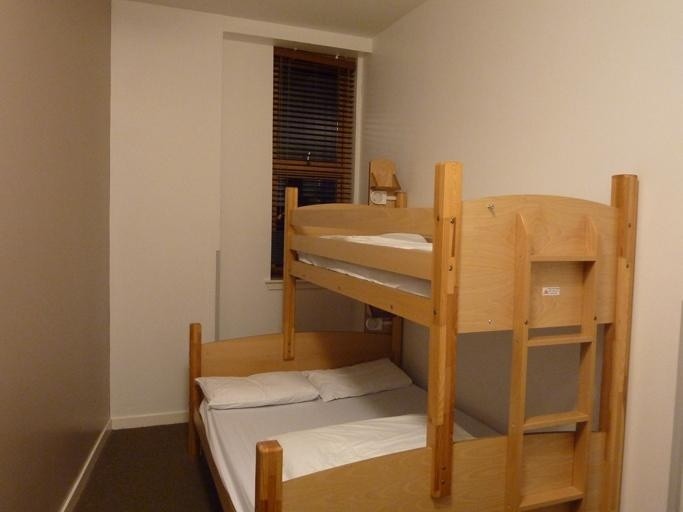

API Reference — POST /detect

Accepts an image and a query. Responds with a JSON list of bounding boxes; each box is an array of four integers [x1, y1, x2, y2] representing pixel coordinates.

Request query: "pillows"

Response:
[[192, 369, 316, 410], [312, 355, 414, 404]]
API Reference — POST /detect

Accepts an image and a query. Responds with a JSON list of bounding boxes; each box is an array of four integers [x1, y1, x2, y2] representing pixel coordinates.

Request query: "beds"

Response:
[[280, 170, 638, 326], [187, 328, 632, 512]]
